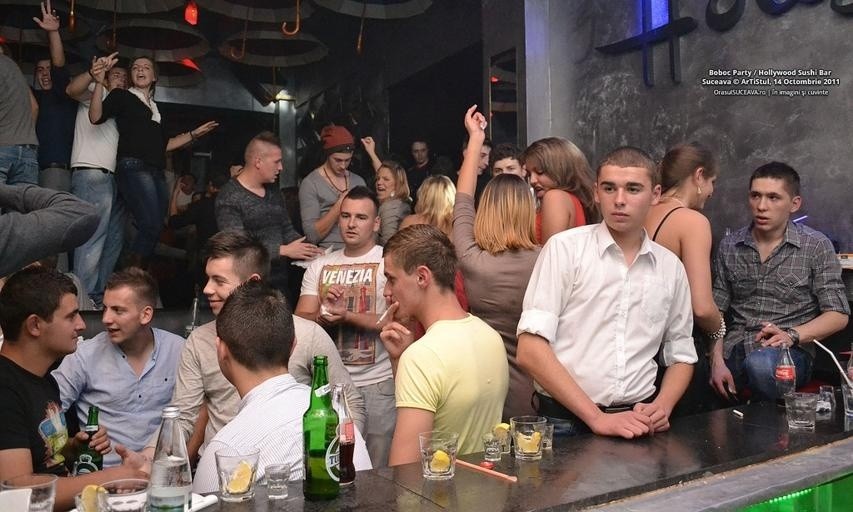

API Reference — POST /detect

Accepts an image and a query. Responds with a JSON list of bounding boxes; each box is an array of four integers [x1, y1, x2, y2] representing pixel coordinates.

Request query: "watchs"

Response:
[[783, 329, 800, 350]]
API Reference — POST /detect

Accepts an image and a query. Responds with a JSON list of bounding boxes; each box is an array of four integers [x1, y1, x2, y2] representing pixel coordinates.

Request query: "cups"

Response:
[[97, 477, 152, 511], [419, 430, 459, 480], [483, 415, 555, 461], [784, 368, 853, 438], [265, 463, 290, 501], [214, 446, 261, 502], [1, 472, 59, 512]]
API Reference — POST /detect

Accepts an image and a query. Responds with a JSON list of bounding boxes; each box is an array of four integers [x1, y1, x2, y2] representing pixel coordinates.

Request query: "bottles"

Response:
[[148, 404, 194, 512], [74, 407, 103, 475], [303, 352, 357, 498], [775, 342, 797, 407]]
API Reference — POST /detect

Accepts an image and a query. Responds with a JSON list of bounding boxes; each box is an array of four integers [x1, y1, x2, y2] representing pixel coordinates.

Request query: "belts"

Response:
[[539, 399, 653, 421], [16, 144, 36, 150], [38, 163, 69, 171], [70, 167, 114, 174]]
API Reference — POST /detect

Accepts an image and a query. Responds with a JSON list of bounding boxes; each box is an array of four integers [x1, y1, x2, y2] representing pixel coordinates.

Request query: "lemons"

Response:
[[493, 423, 510, 435], [517, 431, 541, 451], [81, 485, 106, 512], [430, 450, 450, 472], [225, 459, 253, 495]]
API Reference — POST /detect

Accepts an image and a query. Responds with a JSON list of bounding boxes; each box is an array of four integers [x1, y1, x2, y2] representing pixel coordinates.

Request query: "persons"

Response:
[[2, 0, 526, 512], [709, 160, 852, 410], [523, 136, 604, 248], [452, 102, 546, 429], [644, 141, 726, 420], [514, 146, 700, 441]]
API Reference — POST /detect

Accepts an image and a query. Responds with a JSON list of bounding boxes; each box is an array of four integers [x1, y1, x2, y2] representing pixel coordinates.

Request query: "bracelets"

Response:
[[706, 311, 726, 341]]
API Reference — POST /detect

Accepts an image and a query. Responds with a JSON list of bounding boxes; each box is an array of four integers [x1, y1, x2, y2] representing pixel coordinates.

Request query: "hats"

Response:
[[320, 125, 355, 155]]
